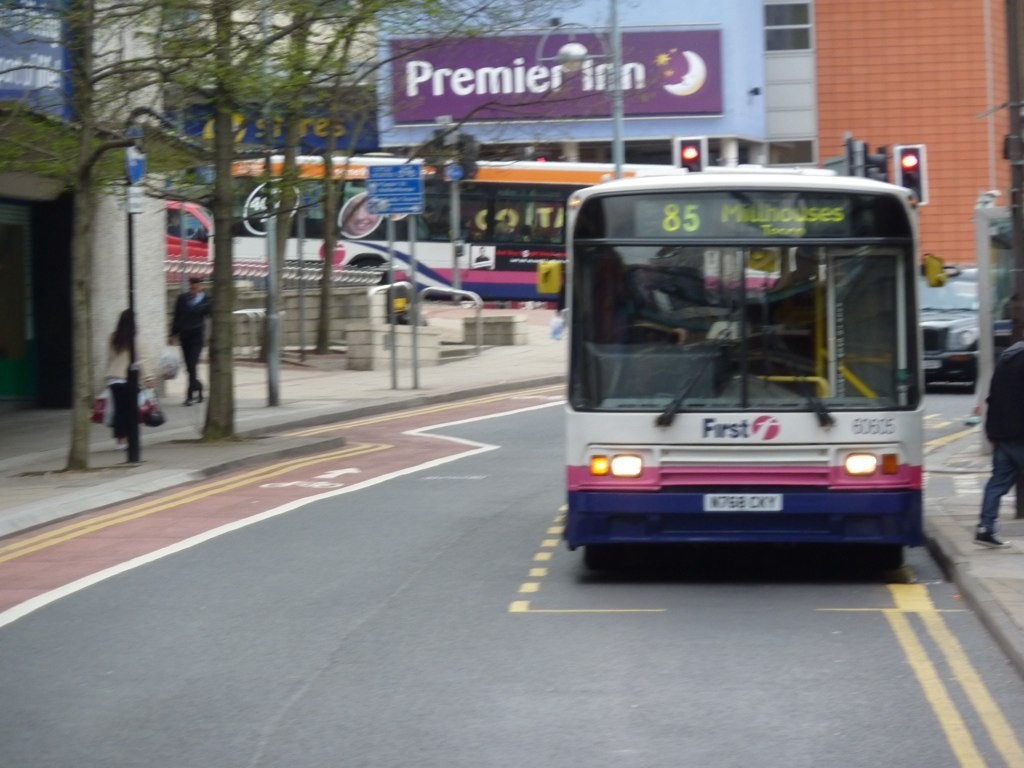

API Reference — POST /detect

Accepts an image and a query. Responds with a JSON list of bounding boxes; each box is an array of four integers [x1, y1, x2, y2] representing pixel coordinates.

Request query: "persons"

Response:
[[103, 308, 155, 450], [166, 276, 213, 406], [617, 245, 815, 345], [973, 317, 1024, 548]]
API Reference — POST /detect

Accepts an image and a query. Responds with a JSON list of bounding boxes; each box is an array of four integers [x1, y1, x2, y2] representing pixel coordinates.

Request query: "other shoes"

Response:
[[115, 443, 129, 450], [186, 398, 194, 406], [973, 532, 1012, 548], [197, 382, 203, 403]]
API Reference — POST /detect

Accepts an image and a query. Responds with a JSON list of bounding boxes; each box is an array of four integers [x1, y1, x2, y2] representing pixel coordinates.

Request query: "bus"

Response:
[[538, 168, 962, 580], [165, 155, 762, 304]]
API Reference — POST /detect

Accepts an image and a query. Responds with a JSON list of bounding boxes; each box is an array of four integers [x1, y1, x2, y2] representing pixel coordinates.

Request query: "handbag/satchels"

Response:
[[137, 388, 166, 426], [155, 346, 180, 378], [550, 316, 565, 340], [89, 388, 115, 426]]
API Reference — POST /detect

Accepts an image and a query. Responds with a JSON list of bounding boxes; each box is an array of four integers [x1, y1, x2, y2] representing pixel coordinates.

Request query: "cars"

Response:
[[913, 265, 1024, 390]]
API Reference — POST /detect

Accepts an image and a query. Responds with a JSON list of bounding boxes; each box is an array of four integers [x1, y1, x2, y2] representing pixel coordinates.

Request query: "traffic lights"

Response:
[[678, 138, 707, 172], [894, 143, 928, 205], [857, 142, 890, 183]]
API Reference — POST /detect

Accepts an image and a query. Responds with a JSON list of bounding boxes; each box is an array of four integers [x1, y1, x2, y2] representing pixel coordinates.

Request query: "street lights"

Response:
[[533, 21, 625, 179]]
